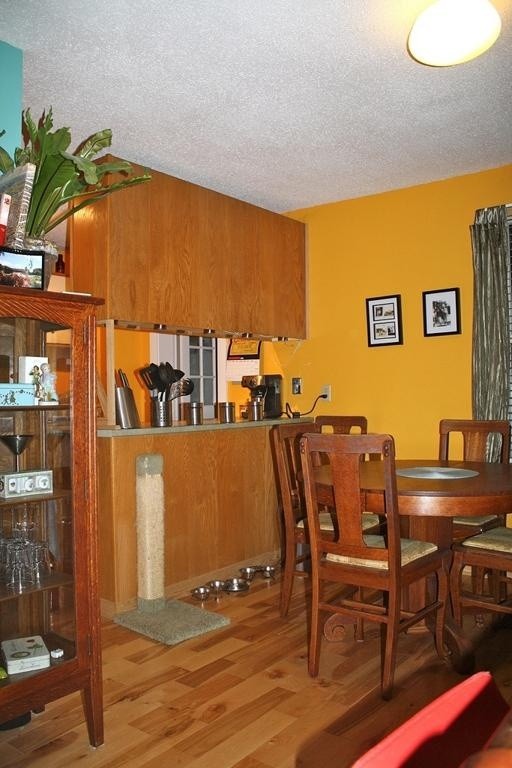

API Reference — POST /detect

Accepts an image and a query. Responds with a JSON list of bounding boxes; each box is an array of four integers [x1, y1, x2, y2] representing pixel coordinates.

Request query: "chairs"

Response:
[[312, 418, 368, 471], [446, 523, 511, 638], [294, 431, 454, 700], [272, 420, 393, 646], [404, 417, 512, 631]]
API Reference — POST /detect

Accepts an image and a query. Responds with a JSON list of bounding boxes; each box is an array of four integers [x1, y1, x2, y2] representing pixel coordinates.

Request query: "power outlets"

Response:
[[322, 385, 330, 401]]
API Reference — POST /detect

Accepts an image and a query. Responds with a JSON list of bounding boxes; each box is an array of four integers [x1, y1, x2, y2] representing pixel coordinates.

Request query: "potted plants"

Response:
[[0, 105, 151, 290]]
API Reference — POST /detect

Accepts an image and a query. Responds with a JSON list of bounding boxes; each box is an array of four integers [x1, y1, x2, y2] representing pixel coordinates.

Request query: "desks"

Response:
[[291, 456, 511, 637]]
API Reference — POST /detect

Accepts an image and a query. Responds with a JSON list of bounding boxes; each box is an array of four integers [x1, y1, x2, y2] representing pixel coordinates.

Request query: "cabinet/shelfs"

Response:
[[1, 284, 104, 747]]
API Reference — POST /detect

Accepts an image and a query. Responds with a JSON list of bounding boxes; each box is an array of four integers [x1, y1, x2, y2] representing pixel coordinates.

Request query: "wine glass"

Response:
[[0, 431, 34, 472]]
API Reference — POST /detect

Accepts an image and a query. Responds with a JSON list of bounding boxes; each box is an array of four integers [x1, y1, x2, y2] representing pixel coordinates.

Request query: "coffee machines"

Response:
[[240, 373, 282, 418]]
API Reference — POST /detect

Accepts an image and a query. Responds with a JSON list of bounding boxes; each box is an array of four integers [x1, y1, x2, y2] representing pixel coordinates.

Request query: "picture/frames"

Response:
[[366, 294, 403, 347], [0, 247, 44, 289], [422, 288, 461, 337]]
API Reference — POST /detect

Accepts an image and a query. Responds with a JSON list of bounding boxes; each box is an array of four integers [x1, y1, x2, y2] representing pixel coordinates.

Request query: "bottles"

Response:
[[56, 254, 65, 274]]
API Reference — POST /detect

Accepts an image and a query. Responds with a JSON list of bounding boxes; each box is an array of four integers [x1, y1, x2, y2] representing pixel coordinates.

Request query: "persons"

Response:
[[29, 365, 42, 398], [41, 362, 59, 402]]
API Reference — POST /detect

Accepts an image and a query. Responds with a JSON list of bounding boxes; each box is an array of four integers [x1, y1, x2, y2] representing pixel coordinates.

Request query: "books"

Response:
[[0, 192, 12, 248], [0, 162, 37, 249]]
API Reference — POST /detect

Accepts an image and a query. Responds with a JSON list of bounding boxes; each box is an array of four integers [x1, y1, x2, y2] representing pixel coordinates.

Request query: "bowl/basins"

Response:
[[191, 567, 276, 600]]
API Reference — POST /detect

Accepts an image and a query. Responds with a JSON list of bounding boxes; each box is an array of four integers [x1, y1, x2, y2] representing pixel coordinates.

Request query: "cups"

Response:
[[247, 401, 263, 421], [150, 402, 171, 426], [1, 535, 52, 591], [116, 388, 141, 430], [219, 402, 236, 424], [183, 402, 204, 425]]
